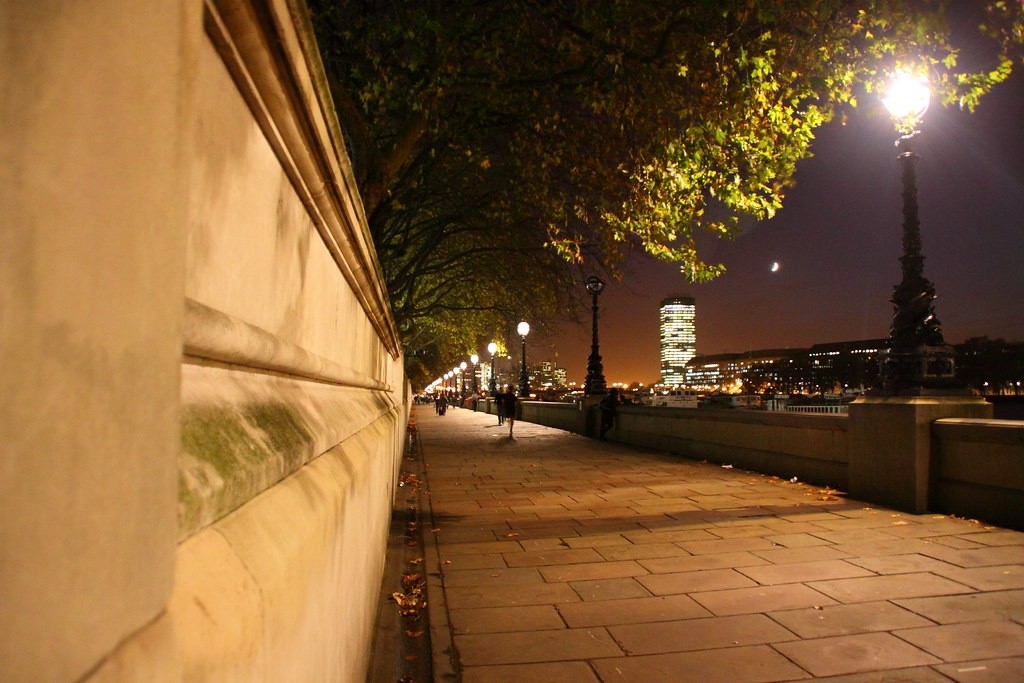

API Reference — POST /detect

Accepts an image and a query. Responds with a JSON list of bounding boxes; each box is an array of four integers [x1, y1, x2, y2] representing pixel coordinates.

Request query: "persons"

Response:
[[494, 385, 522, 438], [413, 388, 481, 416]]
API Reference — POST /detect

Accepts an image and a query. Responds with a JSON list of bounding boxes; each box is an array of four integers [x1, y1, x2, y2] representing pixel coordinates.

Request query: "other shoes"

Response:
[[499, 422, 502, 426]]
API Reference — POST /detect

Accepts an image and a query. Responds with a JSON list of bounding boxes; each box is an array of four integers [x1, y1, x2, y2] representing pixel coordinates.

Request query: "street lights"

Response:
[[470, 354, 479, 395], [878, 65, 973, 397], [460, 361, 468, 398], [517, 322, 530, 399], [453, 367, 460, 398], [448, 371, 454, 396], [443, 373, 449, 396], [583, 273, 607, 396], [487, 342, 497, 398]]
